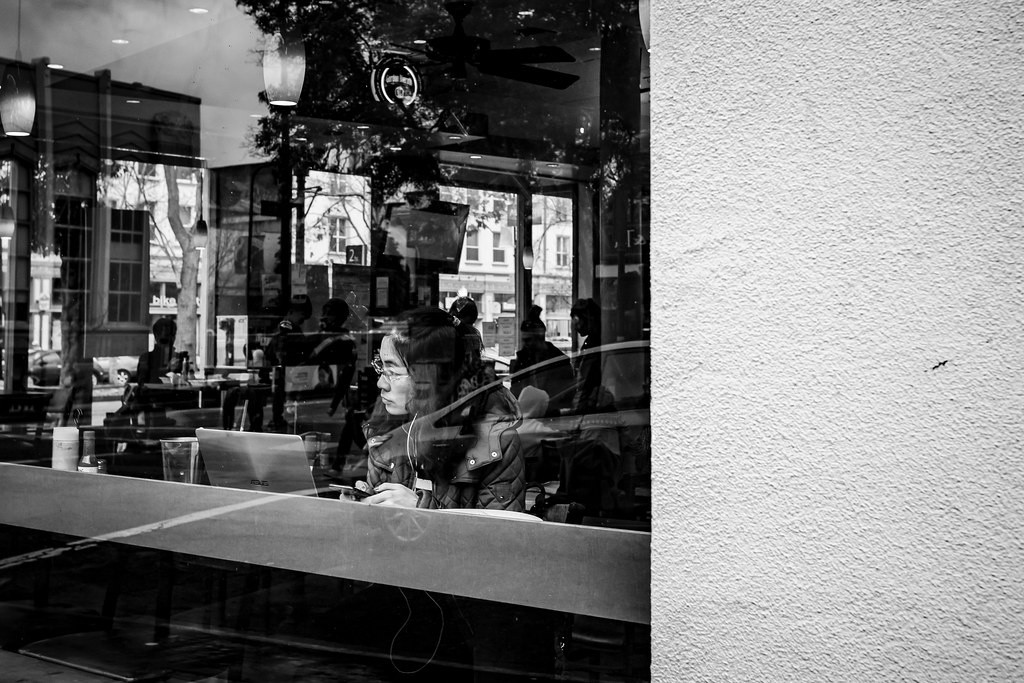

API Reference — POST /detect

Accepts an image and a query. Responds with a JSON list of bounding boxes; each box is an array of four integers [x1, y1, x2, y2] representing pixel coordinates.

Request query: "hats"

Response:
[[521, 306, 546, 332], [324, 297, 349, 320], [290, 294, 313, 312]]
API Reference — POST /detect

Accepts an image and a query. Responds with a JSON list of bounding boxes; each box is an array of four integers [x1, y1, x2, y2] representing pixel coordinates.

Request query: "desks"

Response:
[[0, 378, 365, 507]]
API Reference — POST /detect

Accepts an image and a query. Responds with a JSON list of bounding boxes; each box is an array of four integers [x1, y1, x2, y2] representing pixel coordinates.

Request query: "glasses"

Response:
[[371, 358, 411, 383], [321, 309, 336, 316]]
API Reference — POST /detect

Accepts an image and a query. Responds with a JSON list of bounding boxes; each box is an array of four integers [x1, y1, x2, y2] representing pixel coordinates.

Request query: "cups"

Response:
[[159, 438, 200, 484]]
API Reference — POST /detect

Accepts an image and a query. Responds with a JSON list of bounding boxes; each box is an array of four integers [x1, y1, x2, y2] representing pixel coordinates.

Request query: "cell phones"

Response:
[[329, 484, 372, 499]]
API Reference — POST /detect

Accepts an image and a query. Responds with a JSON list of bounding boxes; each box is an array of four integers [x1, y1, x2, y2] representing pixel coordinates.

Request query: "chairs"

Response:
[[0, 392, 53, 424]]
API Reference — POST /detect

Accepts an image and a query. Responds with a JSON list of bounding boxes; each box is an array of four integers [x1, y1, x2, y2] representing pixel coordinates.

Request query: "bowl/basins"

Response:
[[159, 376, 228, 387]]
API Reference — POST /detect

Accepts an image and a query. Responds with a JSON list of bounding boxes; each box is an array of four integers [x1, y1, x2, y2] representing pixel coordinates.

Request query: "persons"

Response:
[[94, 319, 190, 458], [262, 296, 614, 513]]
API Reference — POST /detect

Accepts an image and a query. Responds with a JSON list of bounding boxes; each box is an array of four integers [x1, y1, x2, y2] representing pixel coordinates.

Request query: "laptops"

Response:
[[194, 429, 319, 498]]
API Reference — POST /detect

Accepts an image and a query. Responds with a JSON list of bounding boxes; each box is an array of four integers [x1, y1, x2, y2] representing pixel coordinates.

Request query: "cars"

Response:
[[0, 347, 141, 390], [546, 335, 572, 351]]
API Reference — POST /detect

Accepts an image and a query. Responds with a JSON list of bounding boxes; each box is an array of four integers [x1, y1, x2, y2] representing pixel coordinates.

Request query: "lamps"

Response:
[[0, 0, 36, 137], [262, 22, 306, 106]]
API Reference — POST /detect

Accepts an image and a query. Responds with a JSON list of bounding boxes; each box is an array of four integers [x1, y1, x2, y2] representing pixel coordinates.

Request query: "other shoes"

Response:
[[324, 470, 344, 480]]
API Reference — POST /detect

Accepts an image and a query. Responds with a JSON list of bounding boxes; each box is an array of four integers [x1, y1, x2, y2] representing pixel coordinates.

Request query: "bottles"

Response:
[[77, 431, 98, 473], [97, 459, 106, 474], [188, 362, 194, 379], [52, 428, 78, 472]]
